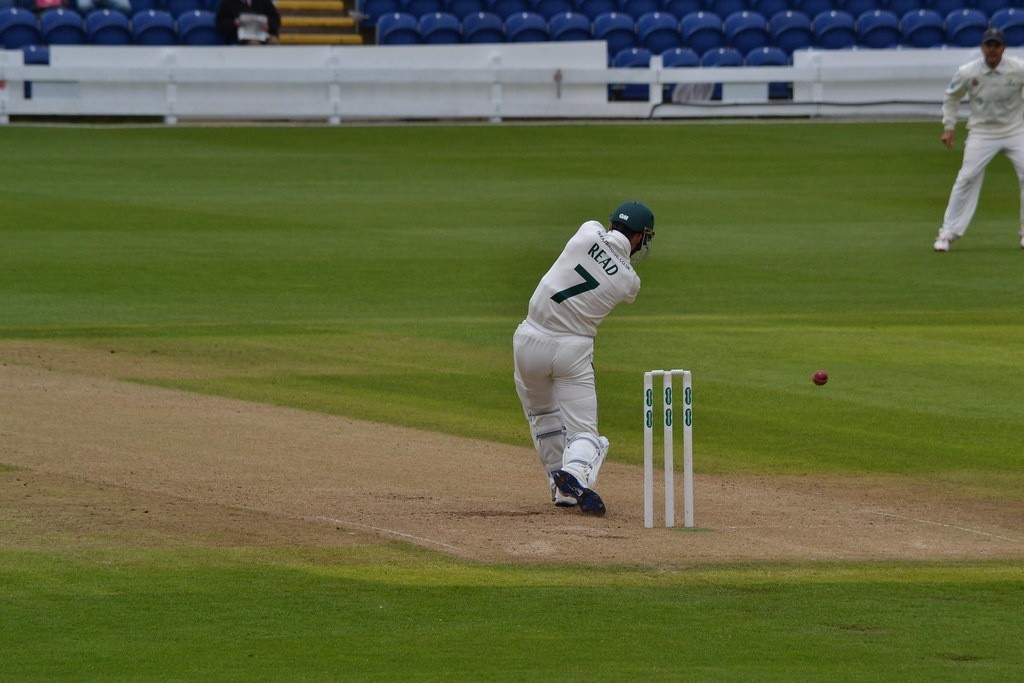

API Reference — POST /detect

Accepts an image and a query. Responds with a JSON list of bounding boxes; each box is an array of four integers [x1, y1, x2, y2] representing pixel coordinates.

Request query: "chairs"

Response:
[[0, 0, 1024, 102]]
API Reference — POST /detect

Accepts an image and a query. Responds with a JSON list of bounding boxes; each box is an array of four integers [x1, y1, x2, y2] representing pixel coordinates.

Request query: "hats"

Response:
[[982, 28, 1004, 45]]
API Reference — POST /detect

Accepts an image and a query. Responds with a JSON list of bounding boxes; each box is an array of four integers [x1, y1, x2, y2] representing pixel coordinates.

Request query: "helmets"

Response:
[[610, 201, 654, 251]]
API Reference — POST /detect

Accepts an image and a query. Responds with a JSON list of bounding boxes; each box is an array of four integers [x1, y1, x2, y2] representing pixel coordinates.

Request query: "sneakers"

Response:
[[934, 236, 952, 250], [554, 485, 577, 507], [554, 468, 606, 517]]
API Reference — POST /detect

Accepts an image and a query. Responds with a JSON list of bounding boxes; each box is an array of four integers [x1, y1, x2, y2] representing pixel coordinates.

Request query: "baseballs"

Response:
[[813, 370, 828, 386]]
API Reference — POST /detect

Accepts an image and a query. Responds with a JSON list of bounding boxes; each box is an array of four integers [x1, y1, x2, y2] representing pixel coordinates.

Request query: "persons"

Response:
[[216, 0, 280, 45], [513, 202, 655, 516], [933, 29, 1024, 253]]
